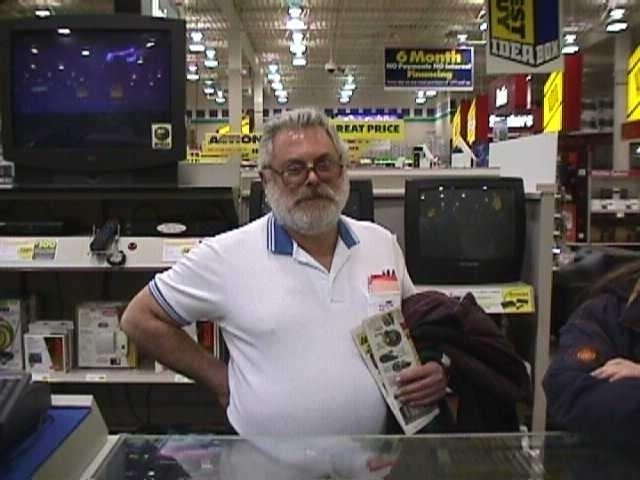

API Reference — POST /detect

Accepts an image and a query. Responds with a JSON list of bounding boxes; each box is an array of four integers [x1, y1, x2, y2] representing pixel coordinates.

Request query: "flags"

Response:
[[381, 44, 477, 93]]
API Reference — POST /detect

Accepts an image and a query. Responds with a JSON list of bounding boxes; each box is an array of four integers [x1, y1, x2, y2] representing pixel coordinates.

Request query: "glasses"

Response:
[[262, 163, 344, 187]]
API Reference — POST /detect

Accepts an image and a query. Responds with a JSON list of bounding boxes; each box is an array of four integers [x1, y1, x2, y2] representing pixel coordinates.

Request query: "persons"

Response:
[[121, 105, 450, 480], [541, 259, 640, 454]]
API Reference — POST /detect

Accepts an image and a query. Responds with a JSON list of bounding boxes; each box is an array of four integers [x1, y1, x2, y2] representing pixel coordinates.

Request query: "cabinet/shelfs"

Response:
[[0, 168, 640, 436]]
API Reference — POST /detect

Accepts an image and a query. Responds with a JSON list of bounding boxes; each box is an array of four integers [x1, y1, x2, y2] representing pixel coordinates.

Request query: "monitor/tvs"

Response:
[[248, 179, 374, 223], [0, 13, 188, 171], [403, 174, 526, 284]]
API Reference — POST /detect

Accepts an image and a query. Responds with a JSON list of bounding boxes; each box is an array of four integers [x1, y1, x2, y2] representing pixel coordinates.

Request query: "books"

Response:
[[0, 290, 223, 375]]
